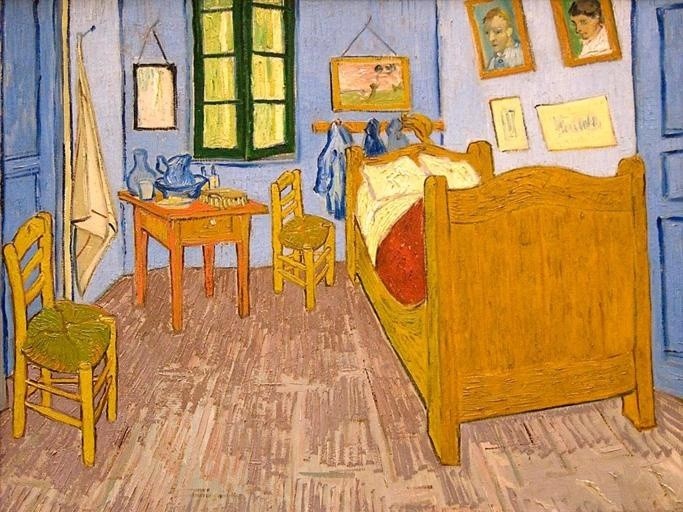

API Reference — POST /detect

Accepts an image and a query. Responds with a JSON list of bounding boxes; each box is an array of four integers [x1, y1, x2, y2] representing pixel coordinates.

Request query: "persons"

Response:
[[566, 0, 614, 62], [481, 5, 525, 74]]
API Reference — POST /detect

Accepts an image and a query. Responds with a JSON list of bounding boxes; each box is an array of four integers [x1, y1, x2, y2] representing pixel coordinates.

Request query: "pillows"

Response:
[[419, 153, 480, 190], [360, 156, 425, 201]]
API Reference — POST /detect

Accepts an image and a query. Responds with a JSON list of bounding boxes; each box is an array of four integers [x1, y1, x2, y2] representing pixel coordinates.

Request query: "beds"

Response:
[[344, 142, 656, 465]]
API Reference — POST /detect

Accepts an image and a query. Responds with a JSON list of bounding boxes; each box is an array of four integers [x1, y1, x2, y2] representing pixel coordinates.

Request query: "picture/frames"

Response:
[[132, 62, 179, 130], [489, 96, 530, 152], [465, 0, 536, 79], [331, 56, 412, 111], [550, 0, 622, 67]]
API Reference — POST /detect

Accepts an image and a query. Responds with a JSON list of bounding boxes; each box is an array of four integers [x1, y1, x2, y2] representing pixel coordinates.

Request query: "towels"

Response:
[[70, 32, 118, 295]]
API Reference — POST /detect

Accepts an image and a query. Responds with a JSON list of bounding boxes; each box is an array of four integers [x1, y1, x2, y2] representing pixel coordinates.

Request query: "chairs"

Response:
[[4, 212, 117, 467], [270, 169, 335, 313]]
